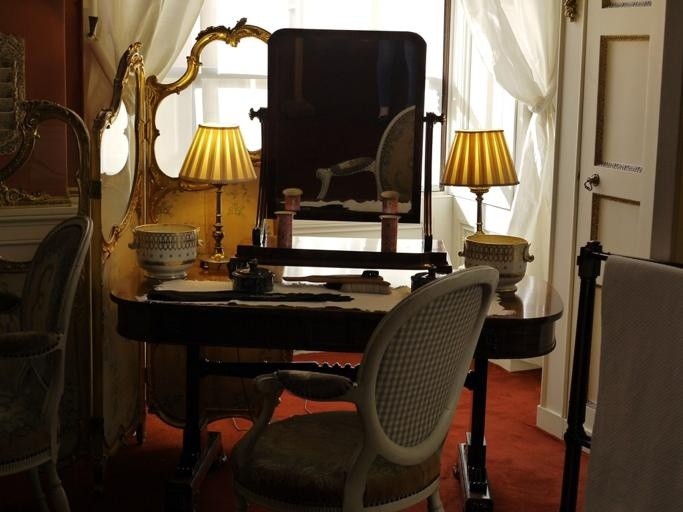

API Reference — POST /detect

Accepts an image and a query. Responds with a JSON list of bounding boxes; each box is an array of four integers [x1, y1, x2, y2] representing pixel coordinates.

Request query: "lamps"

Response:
[[439, 130, 520, 232], [180, 123, 257, 265]]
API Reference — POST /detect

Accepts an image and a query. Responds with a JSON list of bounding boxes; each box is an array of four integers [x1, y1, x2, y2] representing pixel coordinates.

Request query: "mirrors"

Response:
[[266, 28, 427, 224]]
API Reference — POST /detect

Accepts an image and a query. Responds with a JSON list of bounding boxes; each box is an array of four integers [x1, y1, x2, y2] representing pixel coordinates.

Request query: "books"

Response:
[[305, 274, 392, 294]]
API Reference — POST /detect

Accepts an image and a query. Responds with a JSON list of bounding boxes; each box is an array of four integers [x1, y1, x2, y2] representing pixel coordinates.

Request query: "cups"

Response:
[[380, 191, 400, 213], [381, 215, 398, 244], [283, 188, 302, 211], [274, 211, 295, 243]]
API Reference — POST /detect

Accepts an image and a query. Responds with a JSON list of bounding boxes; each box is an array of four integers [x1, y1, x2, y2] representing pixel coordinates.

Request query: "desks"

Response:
[[109, 266, 563, 512]]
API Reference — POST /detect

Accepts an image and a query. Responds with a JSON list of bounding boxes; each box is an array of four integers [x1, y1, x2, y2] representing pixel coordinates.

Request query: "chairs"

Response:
[[0, 216, 93, 511], [232, 267, 499, 512], [314, 95, 415, 202]]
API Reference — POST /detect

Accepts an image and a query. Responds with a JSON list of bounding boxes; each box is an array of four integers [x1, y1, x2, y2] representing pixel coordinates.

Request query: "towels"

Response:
[[585, 256, 683, 512]]
